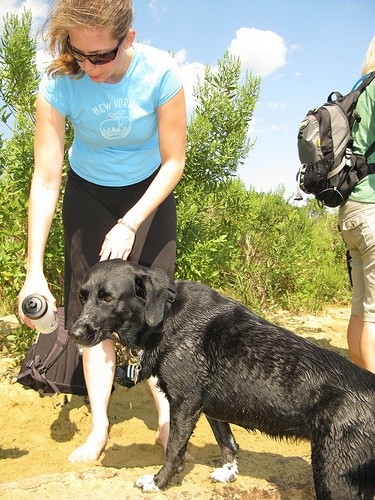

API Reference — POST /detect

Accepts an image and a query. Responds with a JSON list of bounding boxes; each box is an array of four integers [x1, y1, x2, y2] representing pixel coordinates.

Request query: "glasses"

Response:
[[65, 34, 125, 65]]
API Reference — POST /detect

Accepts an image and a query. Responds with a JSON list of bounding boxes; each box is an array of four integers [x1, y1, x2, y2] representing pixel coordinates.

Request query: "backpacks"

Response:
[[298, 72, 375, 208], [18, 307, 115, 397]]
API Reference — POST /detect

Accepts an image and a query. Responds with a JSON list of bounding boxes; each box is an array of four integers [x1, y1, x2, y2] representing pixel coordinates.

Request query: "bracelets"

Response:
[[116, 218, 138, 234]]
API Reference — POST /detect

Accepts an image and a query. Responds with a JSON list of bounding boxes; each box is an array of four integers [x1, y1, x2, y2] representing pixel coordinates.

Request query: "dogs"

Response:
[[68, 259, 375, 500]]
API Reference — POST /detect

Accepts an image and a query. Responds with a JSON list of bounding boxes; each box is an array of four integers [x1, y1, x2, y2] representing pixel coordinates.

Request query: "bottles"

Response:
[[22, 295, 58, 334]]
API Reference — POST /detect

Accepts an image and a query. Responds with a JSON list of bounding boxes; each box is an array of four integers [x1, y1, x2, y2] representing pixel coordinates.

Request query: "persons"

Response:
[[338, 33, 375, 374], [18, 0, 196, 465]]
